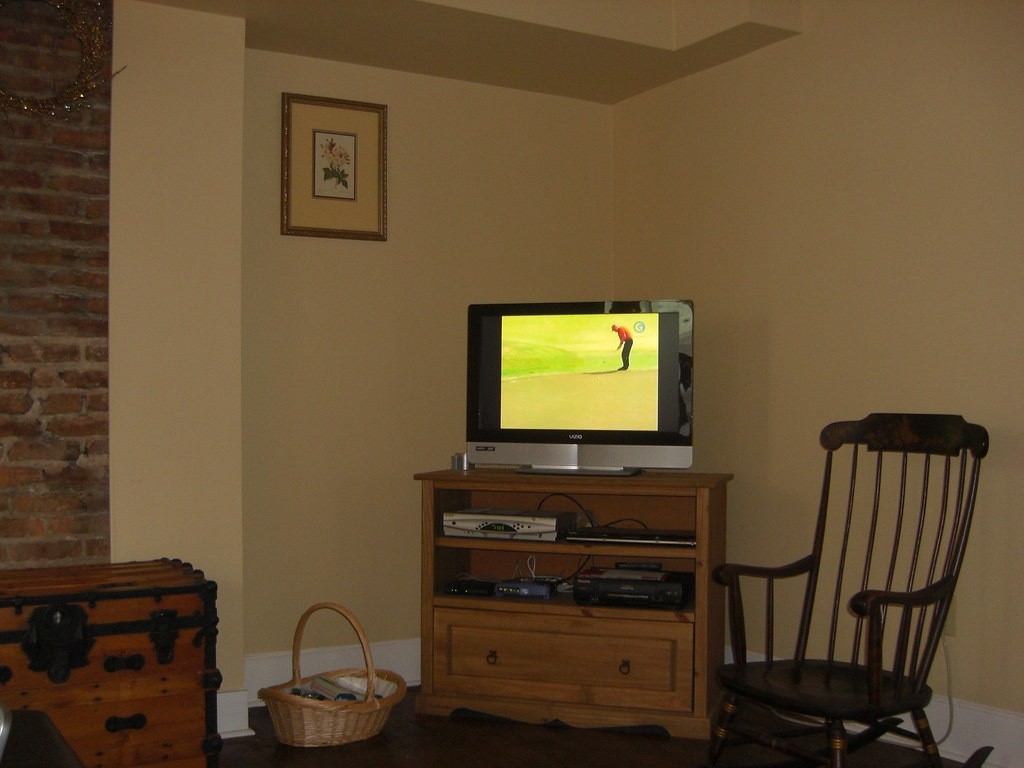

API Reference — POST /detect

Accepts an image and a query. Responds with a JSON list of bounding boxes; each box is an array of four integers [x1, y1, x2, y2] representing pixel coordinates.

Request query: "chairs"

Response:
[[706, 413, 995, 768]]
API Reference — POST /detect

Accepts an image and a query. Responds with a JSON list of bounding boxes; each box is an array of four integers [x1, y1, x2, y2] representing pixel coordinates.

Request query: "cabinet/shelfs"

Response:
[[412, 467, 734, 739]]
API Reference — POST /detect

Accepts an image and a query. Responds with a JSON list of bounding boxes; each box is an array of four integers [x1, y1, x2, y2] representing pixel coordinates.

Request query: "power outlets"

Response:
[[942, 595, 956, 637]]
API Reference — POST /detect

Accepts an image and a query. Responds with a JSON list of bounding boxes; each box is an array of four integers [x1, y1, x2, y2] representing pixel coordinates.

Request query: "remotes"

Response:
[[615, 562, 663, 571]]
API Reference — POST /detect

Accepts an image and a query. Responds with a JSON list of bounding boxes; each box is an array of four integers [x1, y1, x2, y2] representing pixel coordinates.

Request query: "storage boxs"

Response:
[[312, 675, 365, 702], [0, 558, 224, 768]]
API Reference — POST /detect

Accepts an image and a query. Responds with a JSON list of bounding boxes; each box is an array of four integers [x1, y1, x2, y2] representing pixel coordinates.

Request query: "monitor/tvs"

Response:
[[467, 301, 694, 476]]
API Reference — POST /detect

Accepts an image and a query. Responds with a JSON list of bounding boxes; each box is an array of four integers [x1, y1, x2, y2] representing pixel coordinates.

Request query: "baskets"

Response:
[[258, 602, 407, 746]]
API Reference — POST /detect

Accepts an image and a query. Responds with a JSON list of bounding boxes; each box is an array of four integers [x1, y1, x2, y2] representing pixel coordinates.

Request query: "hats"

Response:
[[612, 325, 618, 331]]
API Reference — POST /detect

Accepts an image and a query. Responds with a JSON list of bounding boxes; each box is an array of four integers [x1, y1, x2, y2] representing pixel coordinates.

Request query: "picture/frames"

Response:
[[281, 91, 389, 241]]
